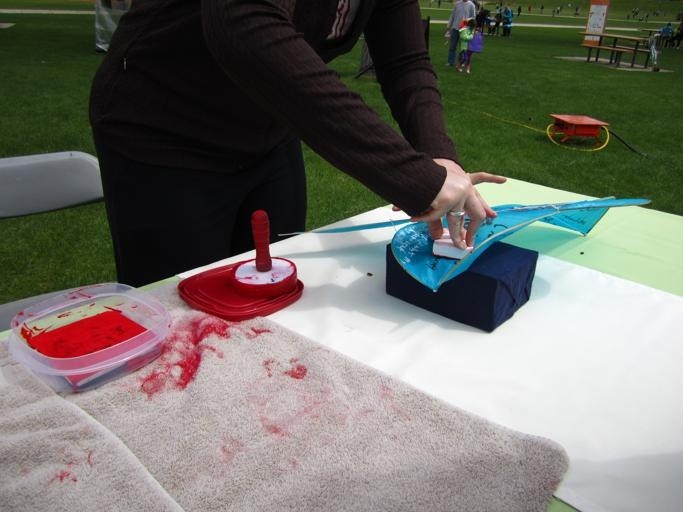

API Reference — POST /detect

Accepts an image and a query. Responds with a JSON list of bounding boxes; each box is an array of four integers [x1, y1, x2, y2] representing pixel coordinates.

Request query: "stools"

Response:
[[0, 150, 105, 219]]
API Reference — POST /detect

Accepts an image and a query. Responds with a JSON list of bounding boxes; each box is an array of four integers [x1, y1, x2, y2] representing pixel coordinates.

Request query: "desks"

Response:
[[0, 169, 683, 510]]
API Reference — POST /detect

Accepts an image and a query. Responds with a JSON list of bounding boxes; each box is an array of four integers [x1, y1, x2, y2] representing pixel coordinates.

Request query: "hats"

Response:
[[466, 19, 477, 26]]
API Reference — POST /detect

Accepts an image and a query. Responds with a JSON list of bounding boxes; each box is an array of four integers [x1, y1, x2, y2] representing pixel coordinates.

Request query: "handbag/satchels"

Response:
[[467, 30, 483, 53]]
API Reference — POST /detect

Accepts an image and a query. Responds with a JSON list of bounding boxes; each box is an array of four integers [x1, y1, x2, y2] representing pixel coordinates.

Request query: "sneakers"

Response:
[[446, 61, 472, 76]]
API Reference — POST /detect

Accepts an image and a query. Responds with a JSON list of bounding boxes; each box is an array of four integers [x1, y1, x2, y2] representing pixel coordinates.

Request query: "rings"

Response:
[[447, 211, 465, 217]]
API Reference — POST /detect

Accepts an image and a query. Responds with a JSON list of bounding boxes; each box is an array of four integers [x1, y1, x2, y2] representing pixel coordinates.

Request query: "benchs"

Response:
[[578, 28, 683, 69]]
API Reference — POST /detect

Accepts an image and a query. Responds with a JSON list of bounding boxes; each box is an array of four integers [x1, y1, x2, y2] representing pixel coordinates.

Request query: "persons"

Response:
[[444, 0, 560, 73], [660, 22, 672, 48], [86, 2, 505, 294], [671, 25, 683, 50]]
